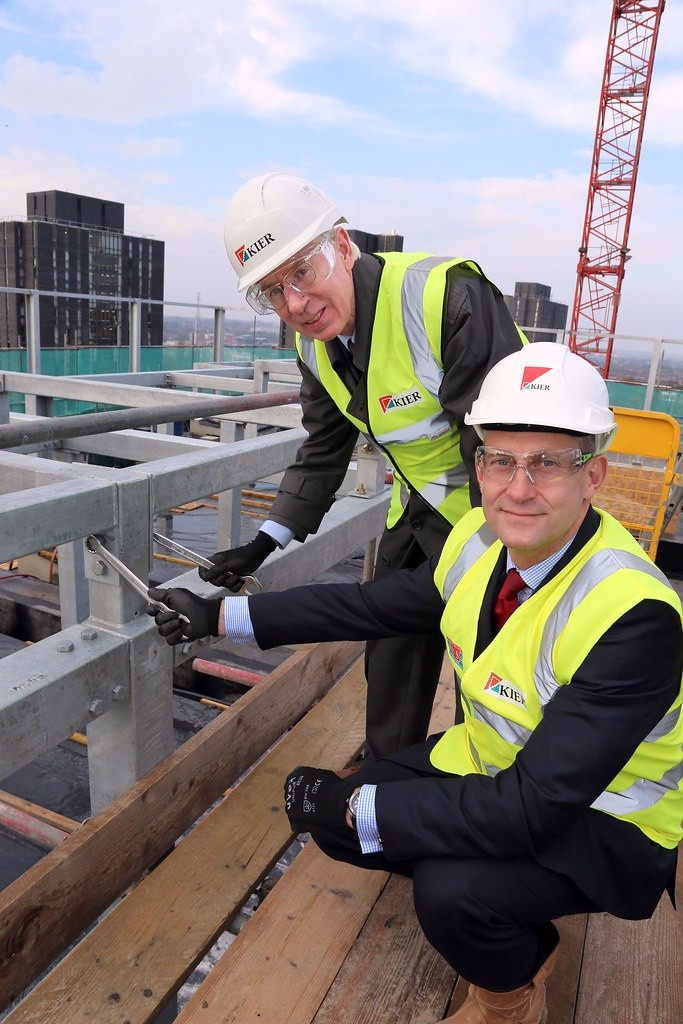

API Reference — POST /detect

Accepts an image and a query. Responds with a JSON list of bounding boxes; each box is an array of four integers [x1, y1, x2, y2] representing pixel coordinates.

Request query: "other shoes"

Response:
[[336, 764, 360, 779]]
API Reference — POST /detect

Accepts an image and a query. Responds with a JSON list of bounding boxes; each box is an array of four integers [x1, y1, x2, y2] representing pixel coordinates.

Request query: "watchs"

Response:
[[346, 787, 361, 830]]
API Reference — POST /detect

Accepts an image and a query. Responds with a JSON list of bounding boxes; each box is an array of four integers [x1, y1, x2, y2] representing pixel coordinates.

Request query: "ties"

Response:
[[494, 568, 525, 629], [344, 340, 354, 358]]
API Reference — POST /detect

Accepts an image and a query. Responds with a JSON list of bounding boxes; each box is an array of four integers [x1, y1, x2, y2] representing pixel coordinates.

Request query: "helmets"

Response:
[[464, 341, 619, 438], [224, 172, 344, 291]]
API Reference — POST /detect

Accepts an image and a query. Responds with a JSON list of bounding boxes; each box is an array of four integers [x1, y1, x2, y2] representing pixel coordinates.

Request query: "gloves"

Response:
[[199, 530, 276, 593], [145, 584, 225, 645], [283, 766, 354, 833]]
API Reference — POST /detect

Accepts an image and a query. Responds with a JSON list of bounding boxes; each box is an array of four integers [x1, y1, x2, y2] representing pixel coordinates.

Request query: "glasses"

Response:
[[245, 235, 336, 315], [254, 236, 332, 309], [475, 440, 591, 485]]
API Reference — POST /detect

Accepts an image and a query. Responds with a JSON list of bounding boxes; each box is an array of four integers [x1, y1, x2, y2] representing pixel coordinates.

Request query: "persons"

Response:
[[145, 342, 683, 1024], [196, 170, 532, 766]]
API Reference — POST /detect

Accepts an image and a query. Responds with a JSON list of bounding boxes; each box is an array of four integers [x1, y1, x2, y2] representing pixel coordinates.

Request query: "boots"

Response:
[[432, 945, 562, 1024]]
